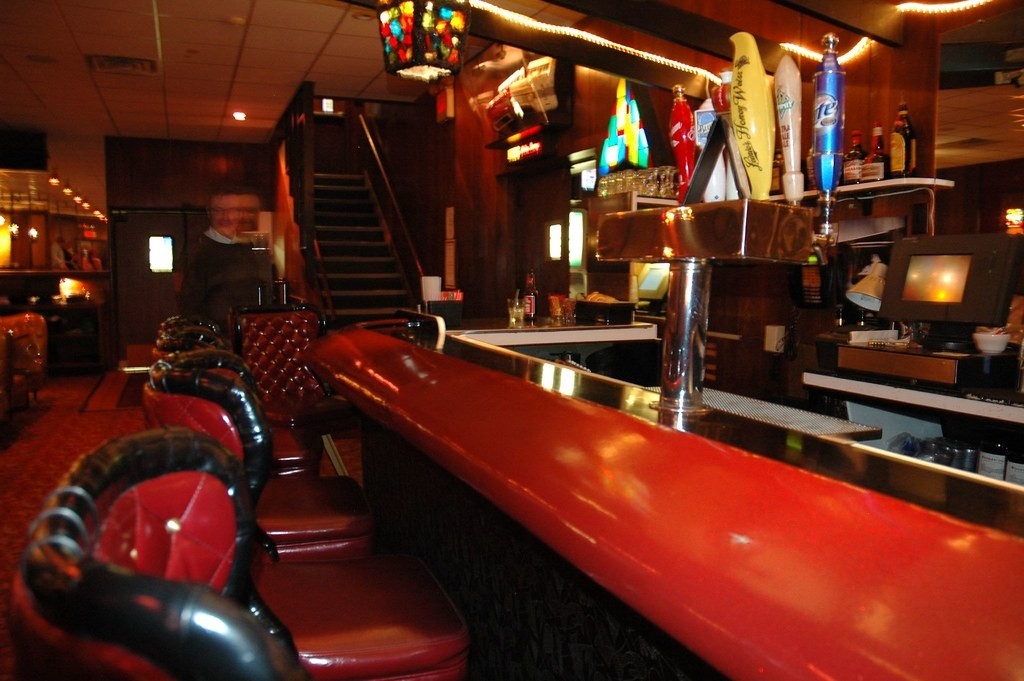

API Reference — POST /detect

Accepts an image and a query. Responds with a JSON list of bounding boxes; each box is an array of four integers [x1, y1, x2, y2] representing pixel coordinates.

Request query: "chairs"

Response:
[[0, 310, 471, 681]]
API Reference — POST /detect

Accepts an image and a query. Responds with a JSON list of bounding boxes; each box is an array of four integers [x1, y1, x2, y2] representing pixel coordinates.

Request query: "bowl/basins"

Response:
[[973, 331, 1011, 354]]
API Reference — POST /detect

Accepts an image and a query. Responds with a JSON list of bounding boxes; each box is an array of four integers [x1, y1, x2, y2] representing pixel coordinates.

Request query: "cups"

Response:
[[598, 165, 678, 199], [507, 298, 525, 329]]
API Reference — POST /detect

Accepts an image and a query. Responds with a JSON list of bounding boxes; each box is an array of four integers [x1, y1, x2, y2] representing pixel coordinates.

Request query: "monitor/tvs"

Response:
[[901, 254, 971, 302]]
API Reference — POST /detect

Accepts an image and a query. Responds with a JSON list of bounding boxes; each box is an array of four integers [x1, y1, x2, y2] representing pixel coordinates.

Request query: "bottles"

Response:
[[522, 277, 537, 327], [842, 130, 868, 184], [834, 303, 845, 327], [890, 101, 918, 178], [806, 141, 818, 190], [769, 149, 784, 195], [857, 307, 866, 326], [864, 122, 889, 182]]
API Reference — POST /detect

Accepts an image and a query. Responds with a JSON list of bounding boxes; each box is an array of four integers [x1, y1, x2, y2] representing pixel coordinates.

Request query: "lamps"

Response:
[[373, 0, 471, 86], [845, 263, 890, 312]]
